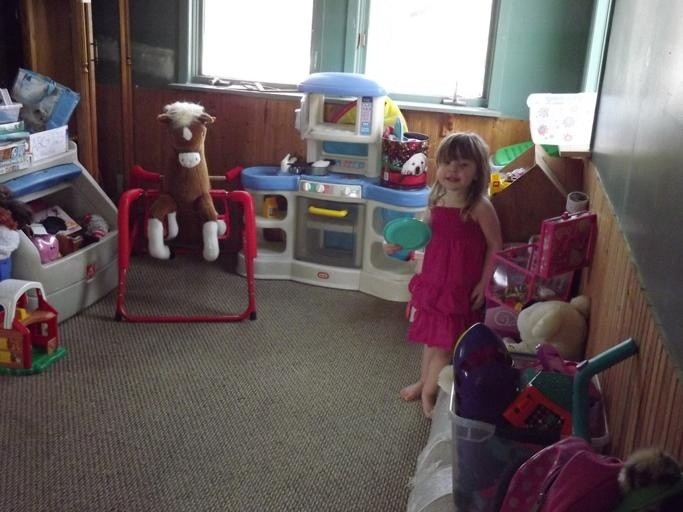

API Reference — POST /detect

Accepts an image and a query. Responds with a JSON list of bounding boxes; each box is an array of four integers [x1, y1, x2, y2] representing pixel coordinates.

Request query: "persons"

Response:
[[382, 132, 503, 420]]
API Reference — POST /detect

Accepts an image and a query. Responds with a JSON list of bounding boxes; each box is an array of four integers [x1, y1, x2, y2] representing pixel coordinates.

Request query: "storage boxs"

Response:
[[487, 139, 584, 242], [448, 362, 614, 512]]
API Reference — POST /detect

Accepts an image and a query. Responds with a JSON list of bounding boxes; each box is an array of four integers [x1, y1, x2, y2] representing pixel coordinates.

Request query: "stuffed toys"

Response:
[[617, 446, 683, 512], [0, 183, 34, 280], [437, 294, 590, 396], [145, 99, 228, 262]]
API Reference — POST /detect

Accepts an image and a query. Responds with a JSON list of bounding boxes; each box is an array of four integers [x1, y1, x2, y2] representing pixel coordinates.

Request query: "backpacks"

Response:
[[500, 436, 625, 512]]
